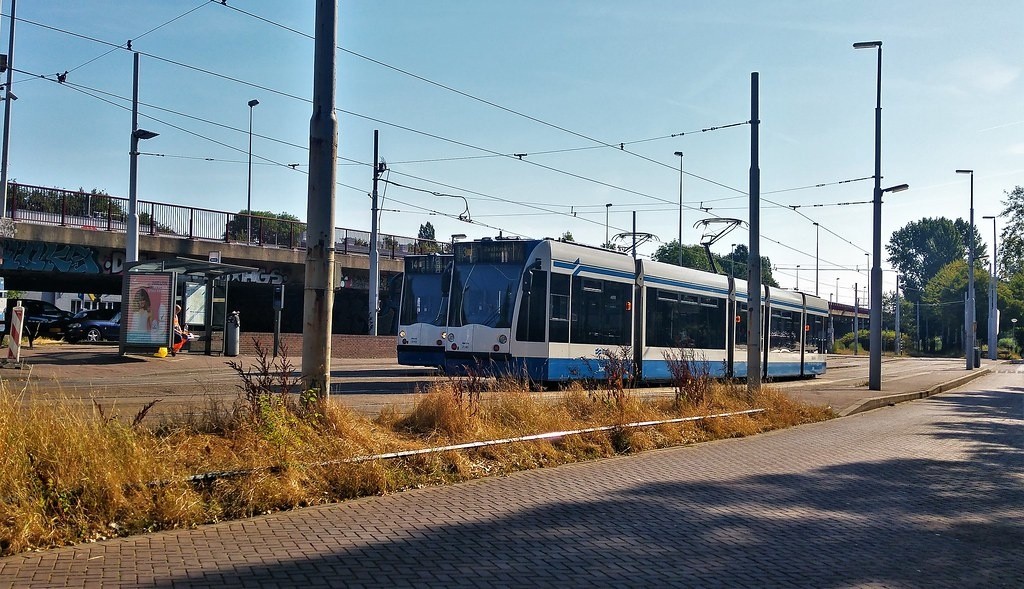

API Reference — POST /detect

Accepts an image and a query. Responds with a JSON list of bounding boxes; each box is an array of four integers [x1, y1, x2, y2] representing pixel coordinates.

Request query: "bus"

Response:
[[449, 216, 829, 379], [396, 253, 452, 366]]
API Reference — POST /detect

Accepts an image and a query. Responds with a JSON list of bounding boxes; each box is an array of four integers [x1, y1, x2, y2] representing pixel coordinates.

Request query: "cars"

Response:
[[68, 308, 123, 342], [5, 298, 70, 340]]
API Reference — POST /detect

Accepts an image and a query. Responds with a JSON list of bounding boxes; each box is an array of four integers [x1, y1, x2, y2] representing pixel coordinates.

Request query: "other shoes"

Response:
[[169, 347, 176, 356]]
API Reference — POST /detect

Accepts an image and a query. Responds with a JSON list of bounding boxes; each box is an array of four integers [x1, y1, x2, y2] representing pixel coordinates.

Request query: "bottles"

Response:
[[184, 323, 188, 334]]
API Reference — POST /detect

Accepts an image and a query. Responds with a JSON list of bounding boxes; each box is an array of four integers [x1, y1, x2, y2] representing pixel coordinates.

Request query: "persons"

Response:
[[130, 289, 153, 333], [171, 304, 188, 357]]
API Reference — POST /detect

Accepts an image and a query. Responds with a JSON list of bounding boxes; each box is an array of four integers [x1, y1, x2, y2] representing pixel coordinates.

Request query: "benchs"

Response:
[[187, 334, 208, 341]]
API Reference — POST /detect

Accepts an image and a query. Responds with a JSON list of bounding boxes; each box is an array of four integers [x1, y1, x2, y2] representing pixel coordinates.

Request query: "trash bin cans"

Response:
[[974, 347, 981, 368], [222, 322, 245, 356]]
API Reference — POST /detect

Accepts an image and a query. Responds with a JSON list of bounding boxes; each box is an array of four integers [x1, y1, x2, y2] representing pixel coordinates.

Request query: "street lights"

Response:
[[981, 216, 998, 360], [836, 277, 840, 303], [674, 151, 685, 265], [1011, 318, 1018, 338], [247, 99, 260, 244], [854, 39, 911, 392], [864, 254, 869, 310], [813, 222, 819, 296], [605, 203, 613, 247], [796, 265, 800, 292], [955, 168, 978, 369], [126, 52, 160, 264]]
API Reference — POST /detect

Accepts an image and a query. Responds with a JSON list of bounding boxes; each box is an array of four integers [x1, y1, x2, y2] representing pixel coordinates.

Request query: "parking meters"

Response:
[[272, 283, 285, 356]]
